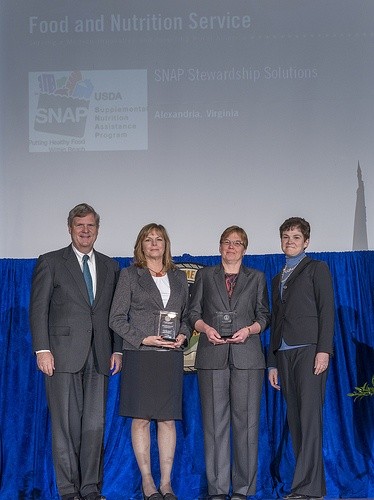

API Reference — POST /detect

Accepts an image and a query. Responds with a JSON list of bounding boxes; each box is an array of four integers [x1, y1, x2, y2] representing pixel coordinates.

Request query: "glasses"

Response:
[[221, 240, 241, 247]]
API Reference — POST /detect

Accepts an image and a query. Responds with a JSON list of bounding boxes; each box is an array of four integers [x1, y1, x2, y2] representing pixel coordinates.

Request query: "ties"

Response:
[[83, 255, 94, 306]]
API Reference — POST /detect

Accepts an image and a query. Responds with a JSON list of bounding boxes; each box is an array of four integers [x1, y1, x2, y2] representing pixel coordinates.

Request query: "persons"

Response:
[[265, 217, 334, 500], [30, 202, 124, 500], [185, 225, 271, 500], [110, 223, 194, 500]]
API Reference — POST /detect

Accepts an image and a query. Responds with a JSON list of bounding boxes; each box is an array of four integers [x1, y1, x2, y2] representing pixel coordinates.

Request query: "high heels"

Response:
[[158, 484, 177, 500], [142, 486, 163, 500]]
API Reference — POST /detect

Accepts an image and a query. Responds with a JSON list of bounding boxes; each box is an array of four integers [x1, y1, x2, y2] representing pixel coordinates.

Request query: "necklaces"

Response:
[[280, 255, 308, 283], [147, 265, 164, 277], [225, 272, 239, 290]]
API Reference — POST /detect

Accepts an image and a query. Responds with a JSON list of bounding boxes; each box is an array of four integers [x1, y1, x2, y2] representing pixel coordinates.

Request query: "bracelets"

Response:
[[247, 327, 251, 336]]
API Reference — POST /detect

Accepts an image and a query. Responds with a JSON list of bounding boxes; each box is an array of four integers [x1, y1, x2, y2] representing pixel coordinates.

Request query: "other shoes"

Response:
[[210, 495, 225, 500], [231, 493, 246, 500], [83, 492, 106, 500], [283, 492, 307, 500], [309, 496, 324, 500], [62, 493, 80, 500]]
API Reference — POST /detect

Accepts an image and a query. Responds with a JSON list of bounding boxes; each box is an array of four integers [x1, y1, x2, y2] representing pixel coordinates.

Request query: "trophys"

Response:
[[155, 309, 179, 341], [214, 311, 236, 338]]
